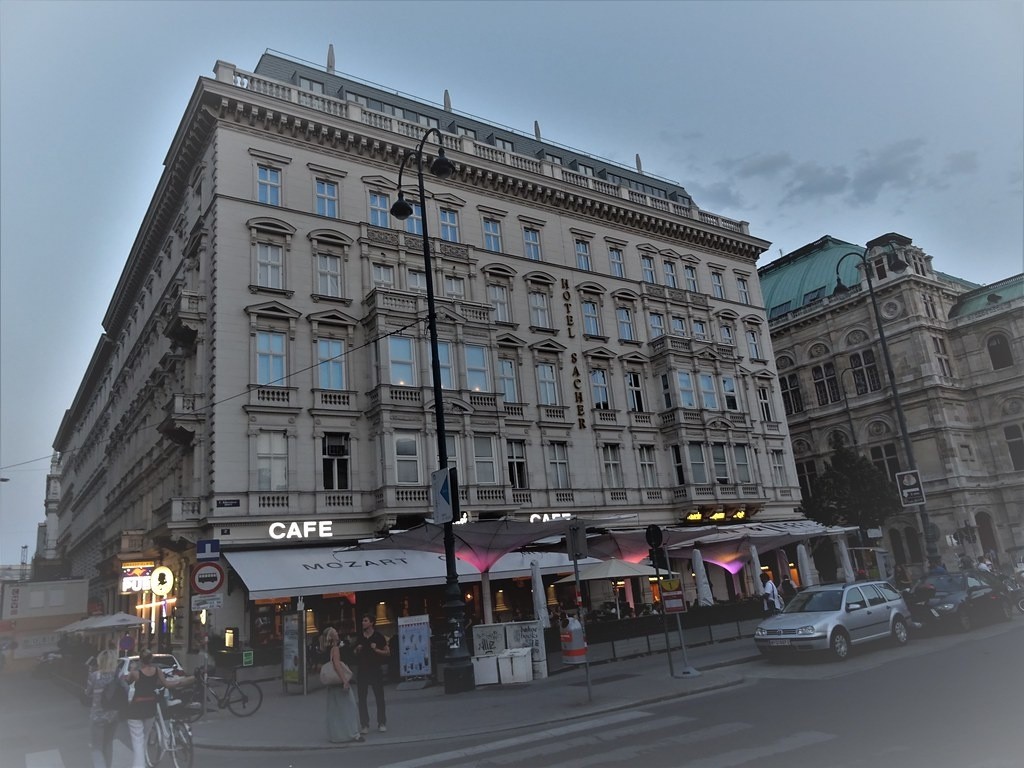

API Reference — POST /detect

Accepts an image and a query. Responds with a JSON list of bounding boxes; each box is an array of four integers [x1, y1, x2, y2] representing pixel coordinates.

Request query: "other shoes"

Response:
[[360, 727, 369, 734], [378, 725, 386, 732], [353, 734, 364, 743]]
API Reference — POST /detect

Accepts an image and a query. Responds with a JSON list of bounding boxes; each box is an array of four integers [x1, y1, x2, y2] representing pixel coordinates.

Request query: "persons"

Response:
[[978, 555, 990, 572], [752, 573, 780, 620], [124, 649, 181, 768], [86, 650, 129, 768], [985, 544, 996, 559], [959, 553, 974, 569], [778, 574, 797, 605], [353, 613, 391, 734], [686, 597, 719, 608], [894, 563, 912, 590], [321, 627, 365, 742], [463, 598, 664, 628]]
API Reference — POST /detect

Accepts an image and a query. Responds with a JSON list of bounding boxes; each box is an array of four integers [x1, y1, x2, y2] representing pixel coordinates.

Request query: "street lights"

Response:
[[833, 242, 944, 568], [388, 127, 478, 696]]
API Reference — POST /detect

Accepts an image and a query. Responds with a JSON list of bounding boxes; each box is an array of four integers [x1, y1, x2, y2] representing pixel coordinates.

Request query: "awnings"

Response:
[[721, 522, 828, 536], [223, 546, 604, 599]]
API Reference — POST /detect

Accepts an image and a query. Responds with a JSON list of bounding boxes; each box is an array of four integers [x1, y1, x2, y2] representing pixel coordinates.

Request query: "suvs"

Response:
[[753, 578, 911, 659]]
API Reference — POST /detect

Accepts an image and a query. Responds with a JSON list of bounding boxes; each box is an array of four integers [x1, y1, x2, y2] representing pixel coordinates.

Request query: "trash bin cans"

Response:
[[470, 654, 499, 686], [559, 617, 587, 664], [497, 647, 533, 684]]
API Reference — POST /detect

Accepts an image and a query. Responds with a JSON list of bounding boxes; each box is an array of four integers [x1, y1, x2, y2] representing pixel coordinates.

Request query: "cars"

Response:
[[906, 568, 1014, 632], [118, 655, 192, 705]]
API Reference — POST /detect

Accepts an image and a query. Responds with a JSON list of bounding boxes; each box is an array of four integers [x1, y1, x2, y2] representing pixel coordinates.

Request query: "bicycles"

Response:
[[149, 684, 196, 768], [188, 666, 265, 718]]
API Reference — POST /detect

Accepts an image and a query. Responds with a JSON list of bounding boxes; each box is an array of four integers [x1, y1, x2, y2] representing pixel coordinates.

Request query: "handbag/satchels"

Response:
[[320, 646, 353, 686]]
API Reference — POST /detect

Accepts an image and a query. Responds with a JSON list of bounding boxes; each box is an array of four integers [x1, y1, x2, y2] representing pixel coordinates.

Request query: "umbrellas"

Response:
[[55, 611, 151, 658], [554, 556, 680, 620]]
